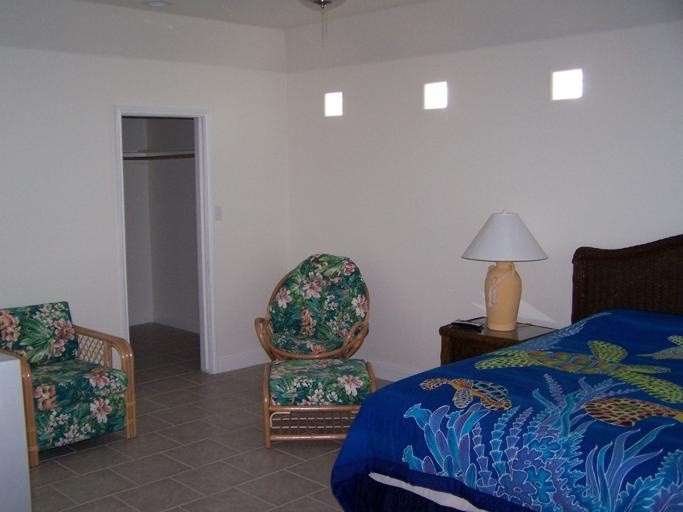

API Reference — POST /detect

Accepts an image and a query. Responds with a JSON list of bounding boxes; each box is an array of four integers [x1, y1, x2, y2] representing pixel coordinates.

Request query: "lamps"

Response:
[[458, 207, 552, 333]]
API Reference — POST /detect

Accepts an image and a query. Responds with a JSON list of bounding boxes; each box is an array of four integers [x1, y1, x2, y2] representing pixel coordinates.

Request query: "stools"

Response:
[[260, 356, 380, 451]]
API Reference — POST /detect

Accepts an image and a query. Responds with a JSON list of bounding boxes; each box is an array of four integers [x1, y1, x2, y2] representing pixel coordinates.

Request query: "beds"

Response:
[[324, 229, 683, 511]]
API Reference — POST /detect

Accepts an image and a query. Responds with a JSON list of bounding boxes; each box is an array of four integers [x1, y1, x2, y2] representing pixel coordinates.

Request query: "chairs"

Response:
[[249, 248, 370, 363], [0, 301, 143, 469]]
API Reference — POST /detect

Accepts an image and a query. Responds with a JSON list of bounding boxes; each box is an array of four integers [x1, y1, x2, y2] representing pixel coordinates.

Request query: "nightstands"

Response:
[[430, 312, 560, 371]]
